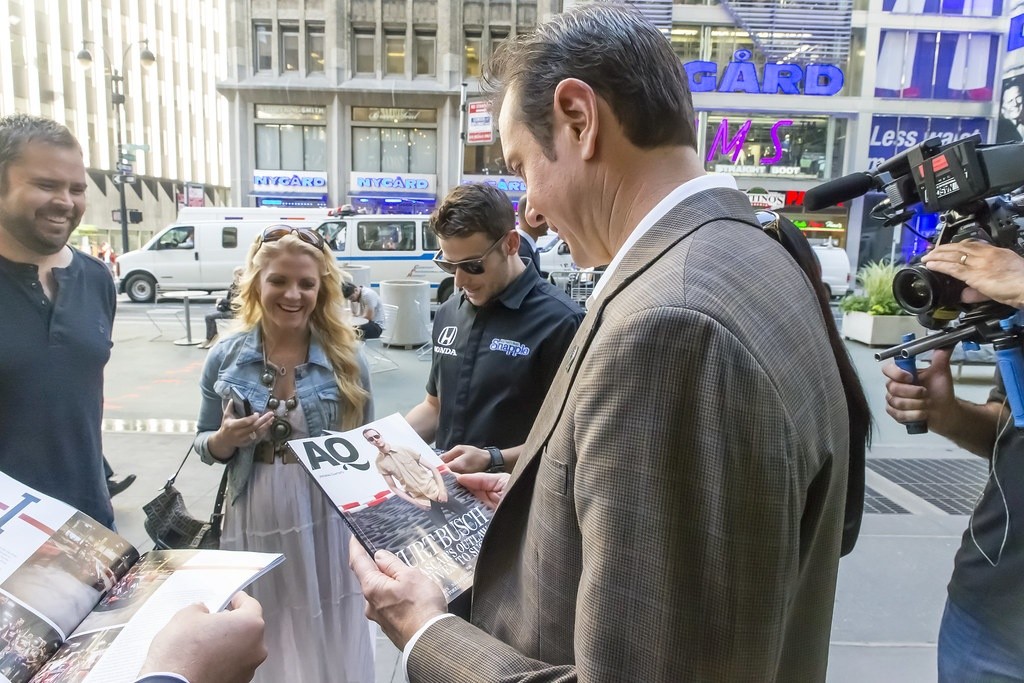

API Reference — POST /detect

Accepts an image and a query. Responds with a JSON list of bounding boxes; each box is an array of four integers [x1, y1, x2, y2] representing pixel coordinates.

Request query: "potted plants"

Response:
[[838, 255, 928, 351]]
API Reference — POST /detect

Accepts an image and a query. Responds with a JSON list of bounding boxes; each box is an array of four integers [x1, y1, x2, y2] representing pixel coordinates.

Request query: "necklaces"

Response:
[[263, 339, 311, 375]]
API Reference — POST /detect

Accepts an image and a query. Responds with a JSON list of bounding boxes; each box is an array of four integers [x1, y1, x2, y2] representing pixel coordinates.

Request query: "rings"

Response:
[[961, 254, 969, 263], [249, 432, 256, 440]]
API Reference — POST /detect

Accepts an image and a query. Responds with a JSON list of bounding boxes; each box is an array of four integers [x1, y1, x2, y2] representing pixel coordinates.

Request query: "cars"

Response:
[[534, 228, 593, 290]]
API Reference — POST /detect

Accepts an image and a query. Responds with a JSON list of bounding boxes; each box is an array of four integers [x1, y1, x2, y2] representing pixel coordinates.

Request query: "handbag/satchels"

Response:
[[142, 486, 220, 550], [217, 298, 229, 312]]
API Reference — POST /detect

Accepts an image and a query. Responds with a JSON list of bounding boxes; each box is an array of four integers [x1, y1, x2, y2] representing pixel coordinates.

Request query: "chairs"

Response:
[[146, 281, 188, 344], [359, 303, 399, 376], [413, 299, 433, 358]]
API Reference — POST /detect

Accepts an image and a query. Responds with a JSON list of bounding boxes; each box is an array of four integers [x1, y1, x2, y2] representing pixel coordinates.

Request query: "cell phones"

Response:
[[230, 386, 251, 417]]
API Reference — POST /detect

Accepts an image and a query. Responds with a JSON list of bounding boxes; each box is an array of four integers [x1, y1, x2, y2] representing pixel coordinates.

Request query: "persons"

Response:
[[1001, 83, 1024, 144], [197, 267, 244, 349], [135, 591, 268, 683], [363, 429, 471, 529], [346, 2, 871, 683], [0, 113, 136, 533], [882, 237, 1024, 683], [104, 457, 137, 501], [89, 240, 119, 284], [514, 196, 550, 277], [399, 182, 585, 475], [729, 134, 805, 172], [191, 225, 375, 683], [342, 282, 385, 339], [755, 212, 870, 557]]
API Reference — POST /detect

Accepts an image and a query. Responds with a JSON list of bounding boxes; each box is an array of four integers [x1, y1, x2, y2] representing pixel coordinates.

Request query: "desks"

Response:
[[347, 316, 369, 326], [162, 290, 209, 346]]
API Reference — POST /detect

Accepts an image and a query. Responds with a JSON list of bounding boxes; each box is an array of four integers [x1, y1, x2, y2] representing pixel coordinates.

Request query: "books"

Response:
[[286, 412, 495, 604], [0, 471, 285, 683]]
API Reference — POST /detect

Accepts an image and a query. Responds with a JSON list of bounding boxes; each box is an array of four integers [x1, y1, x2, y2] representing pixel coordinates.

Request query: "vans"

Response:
[[310, 208, 456, 316], [811, 244, 851, 303], [110, 207, 344, 303]]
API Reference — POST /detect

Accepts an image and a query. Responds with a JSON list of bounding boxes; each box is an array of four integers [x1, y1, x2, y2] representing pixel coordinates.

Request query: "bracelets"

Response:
[[483, 446, 504, 474]]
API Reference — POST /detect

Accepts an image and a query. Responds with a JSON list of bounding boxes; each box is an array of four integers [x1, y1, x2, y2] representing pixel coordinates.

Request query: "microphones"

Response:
[[803, 172, 871, 212]]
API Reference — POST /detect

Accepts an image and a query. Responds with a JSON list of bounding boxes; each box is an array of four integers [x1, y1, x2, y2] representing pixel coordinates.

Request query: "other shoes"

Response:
[[197, 334, 219, 349], [108, 473, 137, 497]]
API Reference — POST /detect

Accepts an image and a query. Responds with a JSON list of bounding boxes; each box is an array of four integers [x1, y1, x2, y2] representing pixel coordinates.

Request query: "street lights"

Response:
[[77, 37, 156, 250]]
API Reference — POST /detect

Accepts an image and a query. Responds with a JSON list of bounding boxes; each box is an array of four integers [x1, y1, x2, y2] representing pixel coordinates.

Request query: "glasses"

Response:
[[367, 435, 380, 442], [432, 233, 507, 275], [754, 208, 783, 242], [255, 224, 327, 255]]
